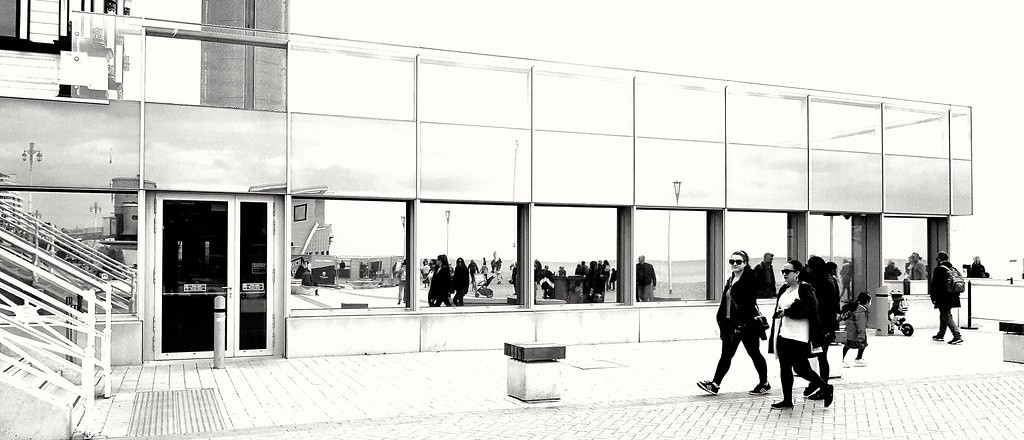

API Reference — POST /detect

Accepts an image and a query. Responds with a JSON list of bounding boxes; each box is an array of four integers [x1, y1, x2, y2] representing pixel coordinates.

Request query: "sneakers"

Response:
[[771, 400, 793, 409], [854, 358, 867, 367], [824, 384, 834, 408], [948, 335, 963, 345], [932, 333, 944, 341], [749, 382, 771, 395], [697, 381, 720, 395], [842, 360, 850, 368]]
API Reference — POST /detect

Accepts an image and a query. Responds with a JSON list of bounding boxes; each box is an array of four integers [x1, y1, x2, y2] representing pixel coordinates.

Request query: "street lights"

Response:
[[673, 180, 681, 205], [445, 211, 450, 258], [89, 201, 101, 247], [21, 141, 43, 229]]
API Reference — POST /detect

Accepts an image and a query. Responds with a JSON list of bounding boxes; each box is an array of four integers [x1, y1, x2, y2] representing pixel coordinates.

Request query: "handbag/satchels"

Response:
[[753, 315, 769, 332]]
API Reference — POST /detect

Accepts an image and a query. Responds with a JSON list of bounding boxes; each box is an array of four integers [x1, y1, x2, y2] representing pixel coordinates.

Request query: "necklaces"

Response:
[[787, 286, 798, 293]]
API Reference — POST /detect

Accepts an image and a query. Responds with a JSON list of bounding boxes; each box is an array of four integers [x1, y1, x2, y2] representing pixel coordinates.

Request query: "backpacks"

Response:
[[939, 265, 965, 293], [841, 300, 860, 321], [898, 296, 909, 312]]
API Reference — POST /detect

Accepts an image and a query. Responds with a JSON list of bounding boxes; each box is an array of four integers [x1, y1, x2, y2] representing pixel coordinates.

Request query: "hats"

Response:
[[937, 250, 949, 262], [888, 259, 896, 266], [857, 292, 872, 305]]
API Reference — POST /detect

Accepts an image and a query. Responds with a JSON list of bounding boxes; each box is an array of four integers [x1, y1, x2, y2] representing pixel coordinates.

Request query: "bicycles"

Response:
[[887, 315, 914, 337]]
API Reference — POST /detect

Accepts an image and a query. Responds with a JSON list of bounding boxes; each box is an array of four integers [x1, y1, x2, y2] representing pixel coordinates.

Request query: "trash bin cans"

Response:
[[553, 275, 607, 302], [360, 265, 368, 279], [875, 284, 888, 336]]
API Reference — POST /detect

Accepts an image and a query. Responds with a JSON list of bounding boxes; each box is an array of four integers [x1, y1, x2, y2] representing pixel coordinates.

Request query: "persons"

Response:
[[5, 223, 128, 280], [970, 256, 986, 278], [301, 254, 657, 308], [840, 258, 852, 299], [753, 253, 777, 298], [930, 251, 964, 345], [768, 260, 834, 409], [888, 289, 905, 329], [805, 256, 872, 367], [697, 250, 772, 395], [884, 253, 928, 280]]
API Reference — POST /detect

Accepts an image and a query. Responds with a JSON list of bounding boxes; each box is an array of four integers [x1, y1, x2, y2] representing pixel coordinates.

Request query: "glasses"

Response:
[[936, 257, 939, 261], [458, 261, 463, 263], [781, 268, 797, 275], [805, 264, 810, 268], [729, 259, 745, 265]]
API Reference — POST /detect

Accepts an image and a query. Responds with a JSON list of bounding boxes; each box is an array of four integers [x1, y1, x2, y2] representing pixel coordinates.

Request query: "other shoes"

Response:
[[804, 382, 819, 396], [398, 300, 401, 305], [807, 390, 824, 400]]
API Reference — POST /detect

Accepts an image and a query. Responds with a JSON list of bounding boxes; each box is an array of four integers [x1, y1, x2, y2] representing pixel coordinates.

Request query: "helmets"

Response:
[[890, 289, 904, 294]]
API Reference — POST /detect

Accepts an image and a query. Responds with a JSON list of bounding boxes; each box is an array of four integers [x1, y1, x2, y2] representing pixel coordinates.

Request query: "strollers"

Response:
[[475, 273, 494, 298]]
